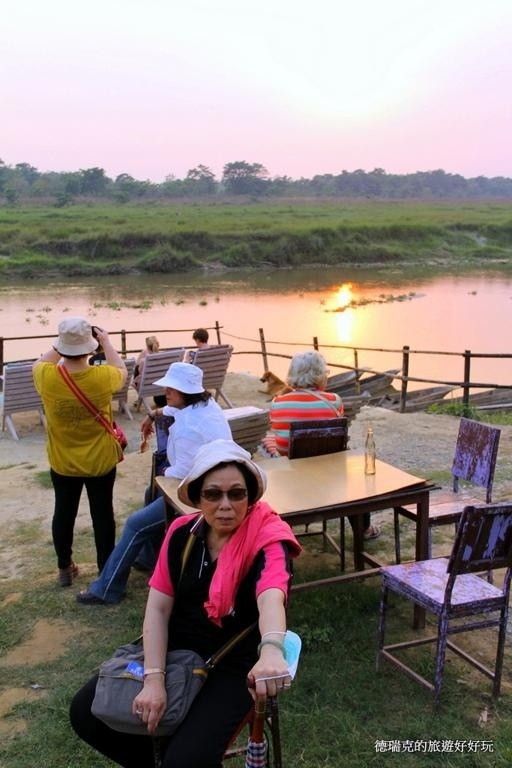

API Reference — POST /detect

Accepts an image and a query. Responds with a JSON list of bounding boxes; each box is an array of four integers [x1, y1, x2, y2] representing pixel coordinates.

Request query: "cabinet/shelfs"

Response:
[[362, 426, 377, 477]]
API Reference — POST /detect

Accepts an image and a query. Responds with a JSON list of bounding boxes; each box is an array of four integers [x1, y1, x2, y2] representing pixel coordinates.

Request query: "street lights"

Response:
[[91, 635, 209, 737], [113, 421, 127, 451]]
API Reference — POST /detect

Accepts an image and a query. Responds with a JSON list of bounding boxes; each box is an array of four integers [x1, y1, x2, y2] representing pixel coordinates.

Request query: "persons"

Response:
[[188, 328, 209, 365], [70, 439, 303, 768], [75, 362, 233, 606], [32, 316, 127, 588], [131, 336, 159, 406], [269, 350, 381, 540], [88, 344, 106, 366]]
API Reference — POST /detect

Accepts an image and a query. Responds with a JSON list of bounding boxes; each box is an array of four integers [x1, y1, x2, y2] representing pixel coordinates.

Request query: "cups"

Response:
[[201, 489, 247, 501], [141, 425, 154, 452]]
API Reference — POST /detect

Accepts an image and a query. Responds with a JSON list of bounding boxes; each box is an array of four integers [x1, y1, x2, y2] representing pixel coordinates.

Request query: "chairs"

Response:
[[364, 526, 380, 540], [59, 562, 78, 586]]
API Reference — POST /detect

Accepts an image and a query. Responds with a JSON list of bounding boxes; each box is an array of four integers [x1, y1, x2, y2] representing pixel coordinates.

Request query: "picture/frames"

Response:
[[147, 448, 443, 628]]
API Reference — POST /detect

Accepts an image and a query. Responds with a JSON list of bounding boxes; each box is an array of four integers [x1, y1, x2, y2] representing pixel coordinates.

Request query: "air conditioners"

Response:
[[257, 371, 294, 405]]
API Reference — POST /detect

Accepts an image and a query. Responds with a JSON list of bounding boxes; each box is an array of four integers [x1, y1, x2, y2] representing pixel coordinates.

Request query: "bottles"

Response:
[[53, 317, 99, 356], [152, 362, 205, 394], [177, 439, 267, 508]]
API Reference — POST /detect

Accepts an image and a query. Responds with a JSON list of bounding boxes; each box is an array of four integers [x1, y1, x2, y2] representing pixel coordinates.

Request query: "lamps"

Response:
[[282, 416, 355, 566], [391, 414, 502, 586], [93, 553, 292, 766], [376, 503, 512, 709]]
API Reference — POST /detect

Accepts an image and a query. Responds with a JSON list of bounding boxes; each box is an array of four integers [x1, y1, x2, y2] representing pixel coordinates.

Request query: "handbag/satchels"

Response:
[[261, 631, 285, 640], [144, 668, 167, 675], [257, 639, 286, 658]]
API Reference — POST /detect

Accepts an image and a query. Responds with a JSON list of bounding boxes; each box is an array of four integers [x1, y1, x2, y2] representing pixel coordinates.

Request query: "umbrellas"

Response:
[[77, 590, 104, 604]]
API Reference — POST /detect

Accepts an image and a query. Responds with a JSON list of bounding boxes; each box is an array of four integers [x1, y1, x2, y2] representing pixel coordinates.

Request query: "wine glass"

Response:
[[284, 684, 292, 689], [136, 710, 143, 715], [277, 686, 283, 689]]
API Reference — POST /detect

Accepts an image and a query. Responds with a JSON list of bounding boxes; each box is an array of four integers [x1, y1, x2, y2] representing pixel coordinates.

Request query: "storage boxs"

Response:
[[149, 398, 365, 459], [126, 344, 238, 420], [3, 356, 59, 439]]
